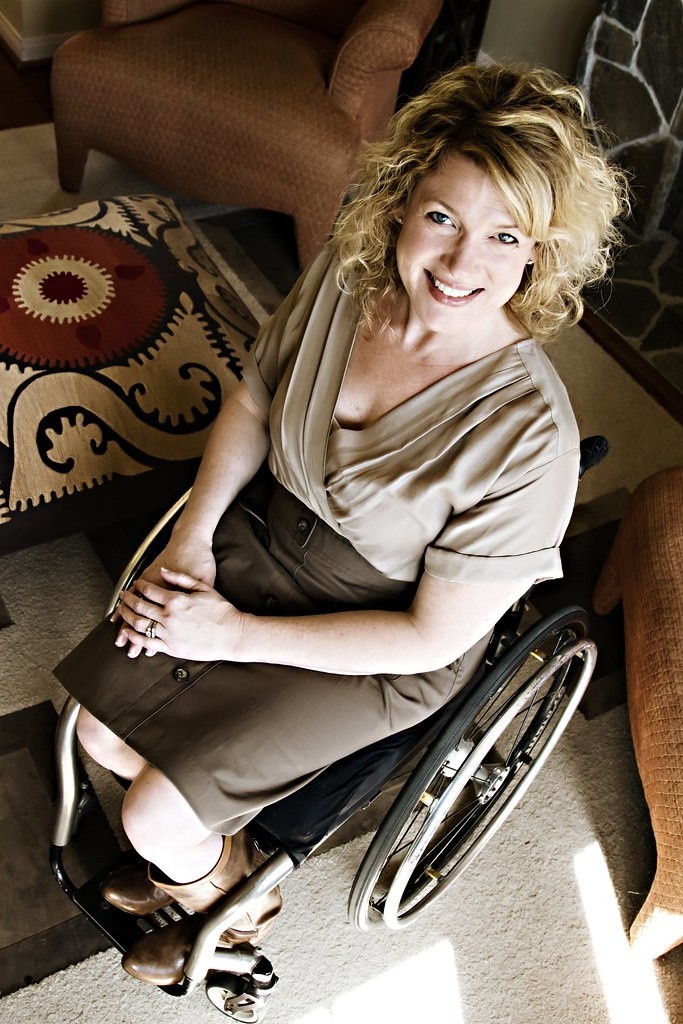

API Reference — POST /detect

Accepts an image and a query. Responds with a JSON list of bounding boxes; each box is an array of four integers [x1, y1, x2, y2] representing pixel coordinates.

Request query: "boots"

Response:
[[122, 836, 283, 985], [102, 770, 176, 915]]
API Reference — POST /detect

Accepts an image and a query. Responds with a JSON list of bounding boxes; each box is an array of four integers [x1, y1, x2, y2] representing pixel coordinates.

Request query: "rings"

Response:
[[145, 620, 156, 638]]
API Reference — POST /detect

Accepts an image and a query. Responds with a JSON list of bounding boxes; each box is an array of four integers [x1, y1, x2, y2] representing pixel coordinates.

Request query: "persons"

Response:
[[77, 59, 630, 986]]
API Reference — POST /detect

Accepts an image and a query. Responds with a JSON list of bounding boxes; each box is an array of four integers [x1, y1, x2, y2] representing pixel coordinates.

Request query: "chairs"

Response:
[[50, 0, 443, 271], [591, 465, 683, 958]]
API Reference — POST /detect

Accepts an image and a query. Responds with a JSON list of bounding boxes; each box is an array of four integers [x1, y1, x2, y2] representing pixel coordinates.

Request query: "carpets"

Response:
[[0, 123, 683, 1024]]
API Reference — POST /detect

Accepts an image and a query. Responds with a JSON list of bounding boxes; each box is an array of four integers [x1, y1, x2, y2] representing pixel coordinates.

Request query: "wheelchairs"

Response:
[[46, 432, 613, 1024]]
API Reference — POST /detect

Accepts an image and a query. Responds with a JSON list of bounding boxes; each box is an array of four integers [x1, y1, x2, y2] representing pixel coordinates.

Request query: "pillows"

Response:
[[0, 194, 260, 558]]
